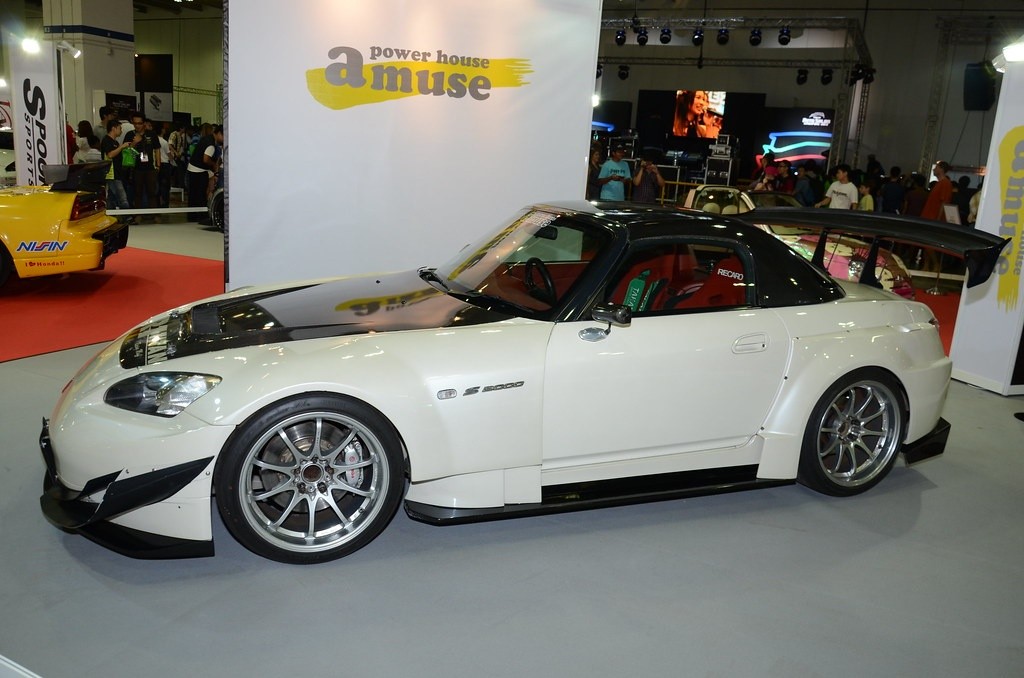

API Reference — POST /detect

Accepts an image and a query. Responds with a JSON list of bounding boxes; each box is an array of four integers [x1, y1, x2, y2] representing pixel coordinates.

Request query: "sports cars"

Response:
[[681, 183, 916, 302], [36, 199, 1012, 566], [0, 160, 129, 291]]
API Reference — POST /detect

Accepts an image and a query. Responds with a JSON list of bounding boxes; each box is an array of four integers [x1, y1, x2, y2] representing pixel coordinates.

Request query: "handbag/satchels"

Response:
[[122, 146, 138, 167]]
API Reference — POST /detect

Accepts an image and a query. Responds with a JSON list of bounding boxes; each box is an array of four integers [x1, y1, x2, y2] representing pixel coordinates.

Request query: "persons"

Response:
[[65, 107, 224, 225], [670, 91, 723, 139], [586, 144, 664, 205], [750, 153, 983, 273]]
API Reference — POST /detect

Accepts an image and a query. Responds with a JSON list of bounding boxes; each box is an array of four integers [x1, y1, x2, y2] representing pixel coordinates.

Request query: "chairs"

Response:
[[609, 242, 746, 311]]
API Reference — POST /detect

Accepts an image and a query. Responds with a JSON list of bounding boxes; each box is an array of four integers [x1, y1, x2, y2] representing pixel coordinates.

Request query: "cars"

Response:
[[0, 126, 17, 183]]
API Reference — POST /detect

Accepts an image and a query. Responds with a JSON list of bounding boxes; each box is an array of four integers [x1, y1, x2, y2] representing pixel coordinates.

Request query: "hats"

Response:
[[866, 154, 876, 160], [765, 154, 775, 160], [935, 161, 949, 172]]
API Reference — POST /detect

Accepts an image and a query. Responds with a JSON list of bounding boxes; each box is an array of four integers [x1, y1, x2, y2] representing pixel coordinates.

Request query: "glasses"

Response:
[[779, 165, 786, 168], [133, 122, 144, 125]]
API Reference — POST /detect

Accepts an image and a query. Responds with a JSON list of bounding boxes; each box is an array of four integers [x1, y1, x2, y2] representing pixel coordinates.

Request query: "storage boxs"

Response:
[[606, 134, 737, 201]]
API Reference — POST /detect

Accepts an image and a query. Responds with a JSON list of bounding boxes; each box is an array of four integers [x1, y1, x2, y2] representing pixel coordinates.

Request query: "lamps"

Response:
[[596, 64, 603, 79], [637, 29, 648, 46], [660, 28, 672, 44], [778, 27, 791, 45], [749, 29, 762, 47], [59, 40, 81, 59], [692, 30, 705, 47], [822, 69, 834, 84], [853, 65, 875, 85], [796, 69, 809, 85], [716, 29, 729, 45], [618, 64, 630, 80], [615, 30, 627, 45]]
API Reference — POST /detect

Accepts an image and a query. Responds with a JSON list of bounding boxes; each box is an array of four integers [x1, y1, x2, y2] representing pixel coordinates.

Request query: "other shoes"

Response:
[[153, 216, 163, 224], [127, 219, 138, 225], [134, 216, 143, 224], [188, 216, 208, 221], [932, 264, 941, 272]]
